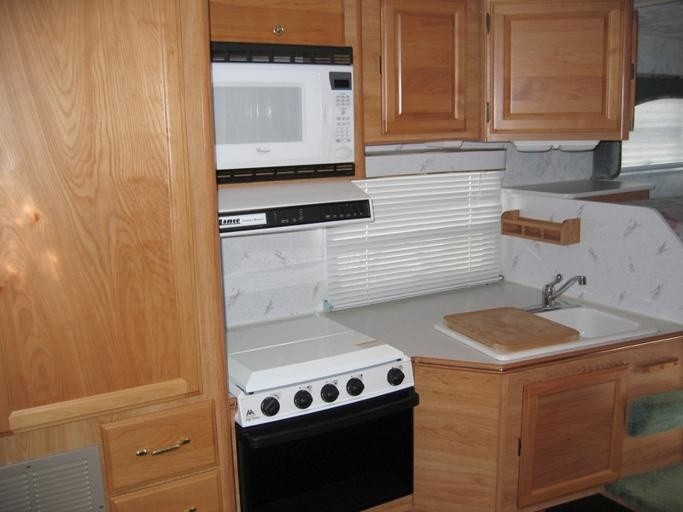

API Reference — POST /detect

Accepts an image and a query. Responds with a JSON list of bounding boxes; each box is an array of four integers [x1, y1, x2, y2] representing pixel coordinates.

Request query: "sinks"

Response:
[[533, 305, 638, 340]]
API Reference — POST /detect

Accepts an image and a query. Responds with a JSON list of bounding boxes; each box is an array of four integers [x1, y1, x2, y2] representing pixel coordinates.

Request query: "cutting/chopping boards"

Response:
[[443, 307, 579, 355]]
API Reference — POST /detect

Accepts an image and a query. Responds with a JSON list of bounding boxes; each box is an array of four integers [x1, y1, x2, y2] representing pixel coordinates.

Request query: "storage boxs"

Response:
[[0, 0, 682, 512]]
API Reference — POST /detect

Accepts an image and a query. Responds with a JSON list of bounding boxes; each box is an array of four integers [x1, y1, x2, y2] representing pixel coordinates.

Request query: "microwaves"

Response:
[[209, 41, 355, 185]]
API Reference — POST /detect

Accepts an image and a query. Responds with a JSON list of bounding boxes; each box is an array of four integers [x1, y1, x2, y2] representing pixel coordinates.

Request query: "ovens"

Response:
[[226, 359, 420, 512]]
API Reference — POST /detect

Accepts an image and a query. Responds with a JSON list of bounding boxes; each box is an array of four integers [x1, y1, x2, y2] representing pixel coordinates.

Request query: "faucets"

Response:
[[541, 271, 589, 307]]
